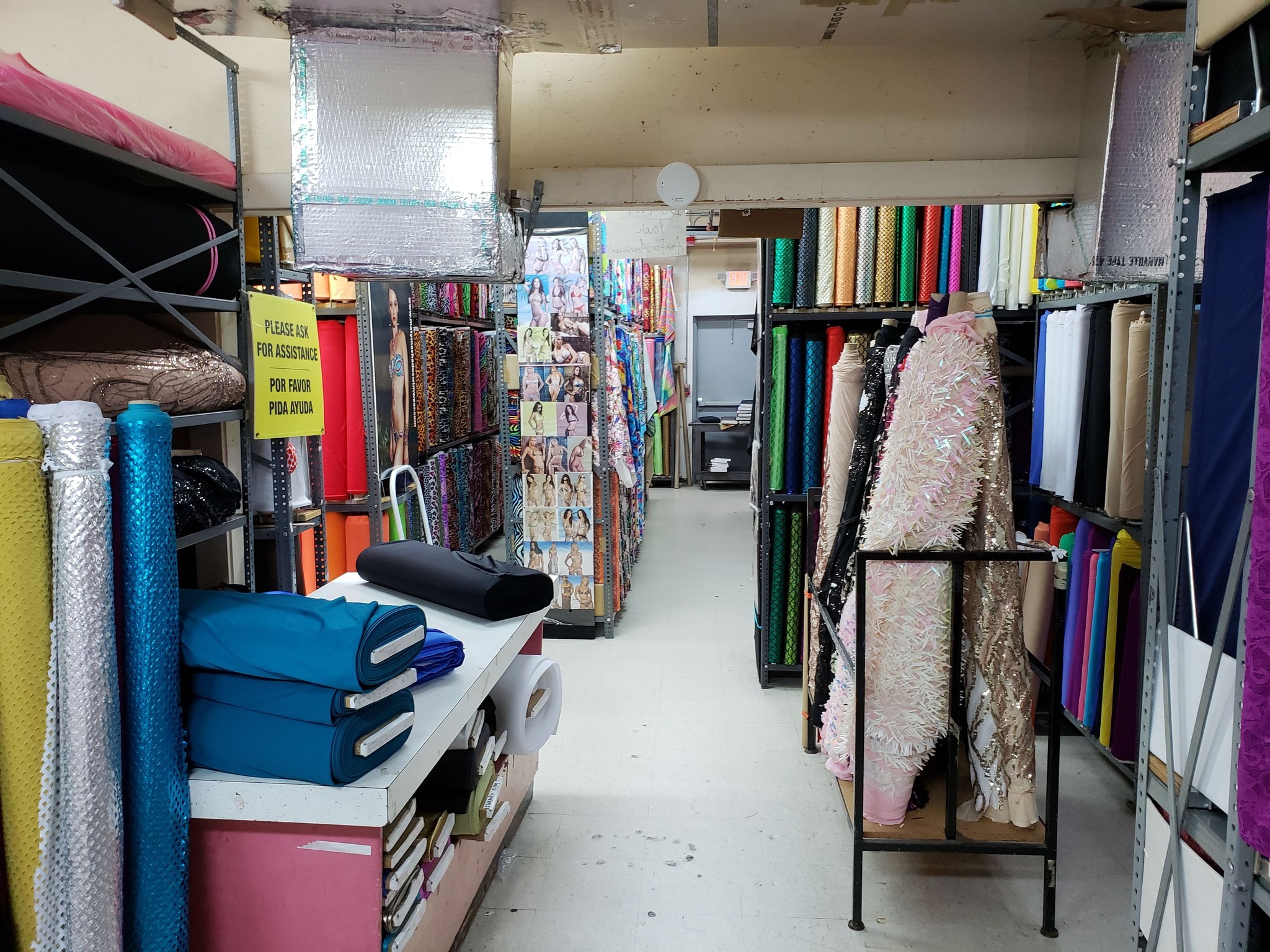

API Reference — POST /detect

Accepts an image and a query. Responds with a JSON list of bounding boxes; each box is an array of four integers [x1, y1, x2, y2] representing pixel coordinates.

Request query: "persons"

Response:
[[560, 509, 590, 541], [531, 237, 586, 274], [522, 363, 544, 401], [550, 277, 586, 313], [522, 277, 549, 327], [558, 474, 588, 507], [551, 336, 590, 364], [558, 403, 579, 437], [548, 543, 558, 574], [522, 328, 551, 363], [569, 438, 591, 472], [527, 509, 556, 541], [565, 542, 583, 576], [521, 436, 545, 474], [564, 366, 584, 402], [529, 402, 544, 435], [545, 366, 564, 402], [560, 576, 593, 609], [527, 474, 555, 507], [553, 313, 590, 336], [546, 438, 567, 474], [528, 541, 544, 572]]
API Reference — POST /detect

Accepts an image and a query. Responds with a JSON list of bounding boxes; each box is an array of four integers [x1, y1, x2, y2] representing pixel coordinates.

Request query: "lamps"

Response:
[[717, 270, 757, 289]]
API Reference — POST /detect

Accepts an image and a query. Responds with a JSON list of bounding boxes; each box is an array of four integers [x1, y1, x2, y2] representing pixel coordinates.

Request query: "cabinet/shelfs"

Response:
[[756, 0, 1270, 952], [0, 0, 675, 952]]
